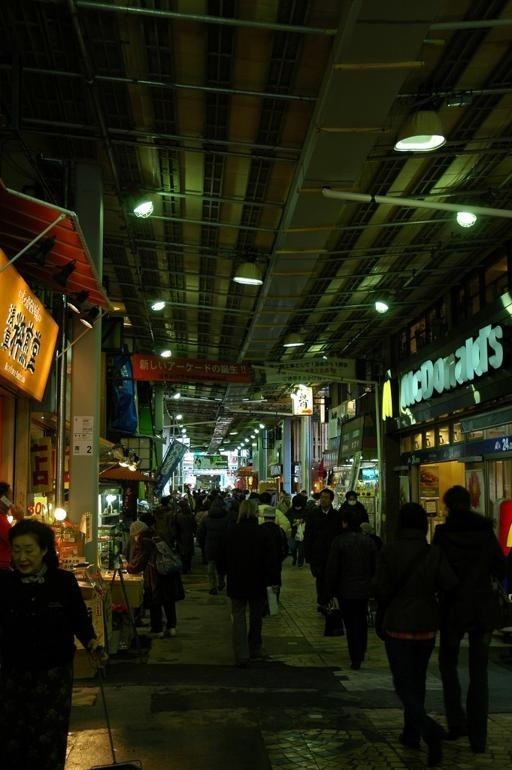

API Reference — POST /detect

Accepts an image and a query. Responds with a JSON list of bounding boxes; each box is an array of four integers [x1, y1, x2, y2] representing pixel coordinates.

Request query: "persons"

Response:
[[225, 498, 282, 660], [434, 486, 512, 755], [327, 509, 377, 671], [124, 522, 177, 636], [138, 487, 259, 595], [249, 489, 382, 637], [1, 520, 107, 768], [125, 488, 137, 523], [375, 503, 465, 746]]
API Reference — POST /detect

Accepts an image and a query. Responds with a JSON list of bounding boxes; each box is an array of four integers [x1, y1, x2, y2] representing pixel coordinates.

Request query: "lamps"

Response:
[[233, 263, 264, 286], [118, 449, 131, 468], [128, 191, 154, 219], [218, 428, 238, 451], [172, 392, 180, 399], [283, 333, 305, 347], [26, 235, 99, 330], [129, 456, 141, 472], [456, 211, 477, 228], [159, 348, 172, 358], [393, 110, 447, 152], [150, 298, 165, 311], [375, 300, 389, 313]]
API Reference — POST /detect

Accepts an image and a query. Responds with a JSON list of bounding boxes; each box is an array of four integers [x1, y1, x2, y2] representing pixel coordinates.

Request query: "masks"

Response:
[[348, 501, 357, 505]]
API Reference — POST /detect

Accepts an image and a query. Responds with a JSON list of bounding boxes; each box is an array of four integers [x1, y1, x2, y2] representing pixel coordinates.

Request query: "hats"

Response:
[[259, 508, 279, 517], [129, 521, 148, 536]]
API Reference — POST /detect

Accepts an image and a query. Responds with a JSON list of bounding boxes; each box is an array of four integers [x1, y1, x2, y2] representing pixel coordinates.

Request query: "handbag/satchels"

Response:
[[324, 608, 345, 635]]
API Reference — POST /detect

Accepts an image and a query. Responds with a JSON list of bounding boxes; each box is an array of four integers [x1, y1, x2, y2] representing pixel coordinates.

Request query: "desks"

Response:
[[103, 570, 144, 650]]
